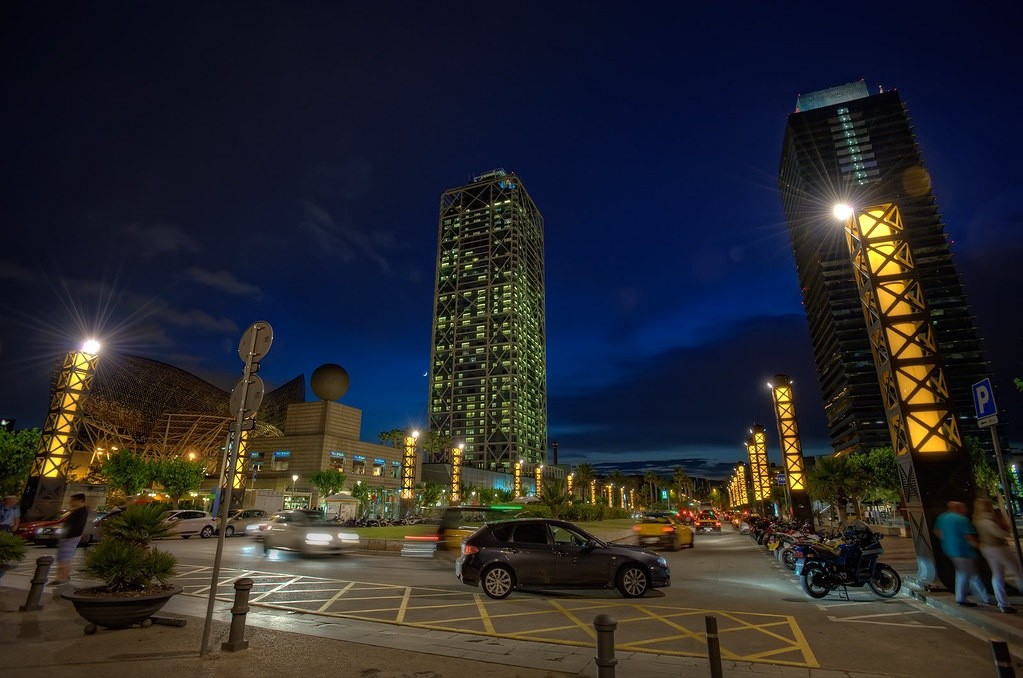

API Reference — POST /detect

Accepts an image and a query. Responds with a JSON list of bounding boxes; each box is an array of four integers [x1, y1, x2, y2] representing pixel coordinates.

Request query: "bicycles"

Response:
[[329, 512, 439, 529]]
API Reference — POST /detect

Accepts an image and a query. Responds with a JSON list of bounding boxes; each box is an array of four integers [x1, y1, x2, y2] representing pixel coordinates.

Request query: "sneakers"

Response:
[[986, 598, 996, 607], [1000, 606, 1017, 614], [48, 579, 69, 587], [957, 601, 978, 607]]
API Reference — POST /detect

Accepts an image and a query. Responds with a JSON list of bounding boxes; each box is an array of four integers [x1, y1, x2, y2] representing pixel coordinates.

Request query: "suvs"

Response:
[[632, 512, 696, 551], [694, 513, 722, 534], [453, 516, 673, 599]]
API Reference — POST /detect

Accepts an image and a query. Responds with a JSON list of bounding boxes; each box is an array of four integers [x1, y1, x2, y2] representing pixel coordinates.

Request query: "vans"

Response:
[[213, 508, 267, 538]]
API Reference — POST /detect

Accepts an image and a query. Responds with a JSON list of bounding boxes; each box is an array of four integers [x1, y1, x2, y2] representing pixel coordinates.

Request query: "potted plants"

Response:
[[59, 500, 188, 636], [0, 529, 27, 579]]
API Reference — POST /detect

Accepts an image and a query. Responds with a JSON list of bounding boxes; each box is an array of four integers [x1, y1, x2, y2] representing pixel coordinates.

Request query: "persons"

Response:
[[933, 501, 990, 608], [972, 499, 1023, 613], [45, 491, 88, 589], [0, 495, 21, 534]]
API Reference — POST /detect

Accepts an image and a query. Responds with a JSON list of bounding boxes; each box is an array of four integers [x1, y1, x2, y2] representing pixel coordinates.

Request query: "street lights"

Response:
[[629, 488, 635, 510], [726, 465, 742, 510], [448, 445, 464, 508], [401, 432, 420, 520], [737, 460, 748, 508], [512, 461, 521, 499], [565, 471, 575, 506], [216, 412, 254, 513], [749, 420, 775, 520], [619, 485, 626, 509], [19, 337, 101, 524], [589, 478, 597, 505], [289, 475, 301, 510], [768, 374, 817, 534], [604, 482, 613, 509], [533, 462, 544, 499], [742, 435, 763, 510], [841, 200, 991, 596]]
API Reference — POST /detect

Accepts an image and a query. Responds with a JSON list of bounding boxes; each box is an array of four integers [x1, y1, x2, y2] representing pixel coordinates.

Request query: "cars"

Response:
[[33, 511, 112, 548], [657, 501, 749, 526], [160, 510, 217, 539], [255, 509, 359, 557], [739, 514, 761, 534], [433, 505, 549, 565], [15, 508, 69, 545]]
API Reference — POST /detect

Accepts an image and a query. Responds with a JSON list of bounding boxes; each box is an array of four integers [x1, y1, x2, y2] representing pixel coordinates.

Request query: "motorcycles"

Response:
[[743, 511, 861, 571], [792, 518, 902, 601]]
